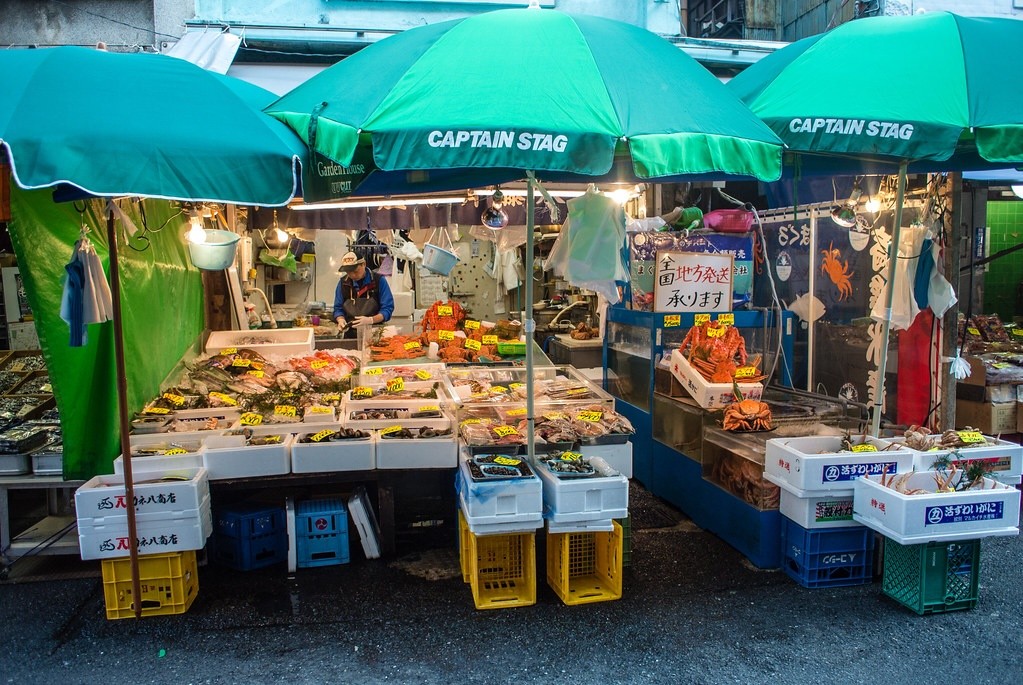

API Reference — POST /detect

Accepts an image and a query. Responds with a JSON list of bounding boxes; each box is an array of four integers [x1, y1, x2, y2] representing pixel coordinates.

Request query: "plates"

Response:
[[545, 459, 596, 477], [473, 454, 523, 477]]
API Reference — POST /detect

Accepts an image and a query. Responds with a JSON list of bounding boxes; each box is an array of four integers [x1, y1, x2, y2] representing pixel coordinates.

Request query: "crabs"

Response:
[[371, 299, 504, 363], [679, 321, 1009, 495]]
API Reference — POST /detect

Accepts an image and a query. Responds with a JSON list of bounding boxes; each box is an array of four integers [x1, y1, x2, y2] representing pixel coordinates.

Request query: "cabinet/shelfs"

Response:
[[254, 261, 312, 285]]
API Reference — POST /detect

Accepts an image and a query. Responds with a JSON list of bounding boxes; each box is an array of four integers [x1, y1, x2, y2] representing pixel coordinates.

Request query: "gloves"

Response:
[[338, 319, 349, 333], [352, 315, 373, 328]]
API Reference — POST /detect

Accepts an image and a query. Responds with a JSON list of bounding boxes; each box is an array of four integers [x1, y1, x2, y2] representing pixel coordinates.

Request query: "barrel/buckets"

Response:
[[185, 229, 241, 271], [422, 243, 461, 276]]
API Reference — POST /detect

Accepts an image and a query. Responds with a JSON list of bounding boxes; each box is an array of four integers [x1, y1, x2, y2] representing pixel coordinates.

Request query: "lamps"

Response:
[[287, 195, 467, 210], [829, 179, 865, 228], [481, 182, 510, 230], [468, 188, 585, 197]]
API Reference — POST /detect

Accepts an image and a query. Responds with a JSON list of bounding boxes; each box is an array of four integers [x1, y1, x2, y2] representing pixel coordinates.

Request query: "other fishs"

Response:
[[94, 337, 636, 487]]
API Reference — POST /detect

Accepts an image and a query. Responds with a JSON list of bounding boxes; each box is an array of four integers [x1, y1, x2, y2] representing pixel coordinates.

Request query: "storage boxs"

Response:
[[72, 276, 634, 618], [762, 385, 1023, 614], [668, 349, 762, 412], [0, 438, 64, 476]]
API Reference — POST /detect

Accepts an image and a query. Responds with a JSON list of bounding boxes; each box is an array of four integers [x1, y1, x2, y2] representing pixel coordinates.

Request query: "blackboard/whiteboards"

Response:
[[653, 250, 734, 313]]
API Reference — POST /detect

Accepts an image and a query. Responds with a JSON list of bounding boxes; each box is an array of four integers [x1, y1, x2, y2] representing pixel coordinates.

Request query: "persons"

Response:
[[333, 251, 394, 339]]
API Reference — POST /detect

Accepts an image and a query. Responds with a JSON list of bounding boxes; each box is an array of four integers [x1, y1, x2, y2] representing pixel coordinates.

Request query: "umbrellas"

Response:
[[262, 0, 790, 467], [728, 7, 1023, 439], [0, 48, 318, 619]]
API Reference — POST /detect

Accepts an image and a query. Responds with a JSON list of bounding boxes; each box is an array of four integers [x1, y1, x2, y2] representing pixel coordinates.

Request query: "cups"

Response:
[[312, 316, 320, 326]]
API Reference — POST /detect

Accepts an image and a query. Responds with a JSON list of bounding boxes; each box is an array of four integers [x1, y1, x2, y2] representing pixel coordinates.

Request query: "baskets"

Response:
[[497, 342, 527, 354]]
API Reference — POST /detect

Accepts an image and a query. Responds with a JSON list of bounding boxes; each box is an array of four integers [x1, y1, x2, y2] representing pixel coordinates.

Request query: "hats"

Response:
[[338, 251, 365, 271]]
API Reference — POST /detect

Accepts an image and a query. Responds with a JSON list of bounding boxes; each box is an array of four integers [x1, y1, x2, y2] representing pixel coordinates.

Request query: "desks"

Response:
[[0, 475, 79, 580], [209, 468, 457, 559]]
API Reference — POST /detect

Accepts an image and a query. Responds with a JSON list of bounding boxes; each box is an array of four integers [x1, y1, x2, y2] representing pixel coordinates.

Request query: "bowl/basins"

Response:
[[532, 303, 545, 309]]
